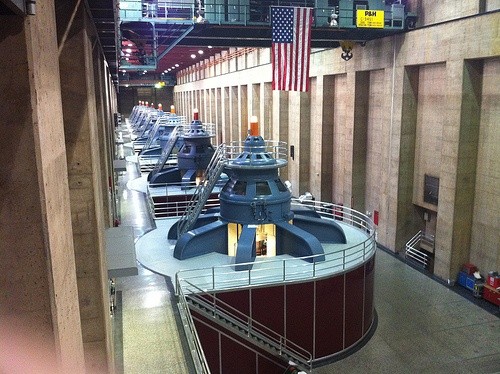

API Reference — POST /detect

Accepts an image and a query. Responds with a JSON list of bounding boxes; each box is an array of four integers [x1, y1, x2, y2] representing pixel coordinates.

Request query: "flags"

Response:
[[266, 7, 314, 93]]
